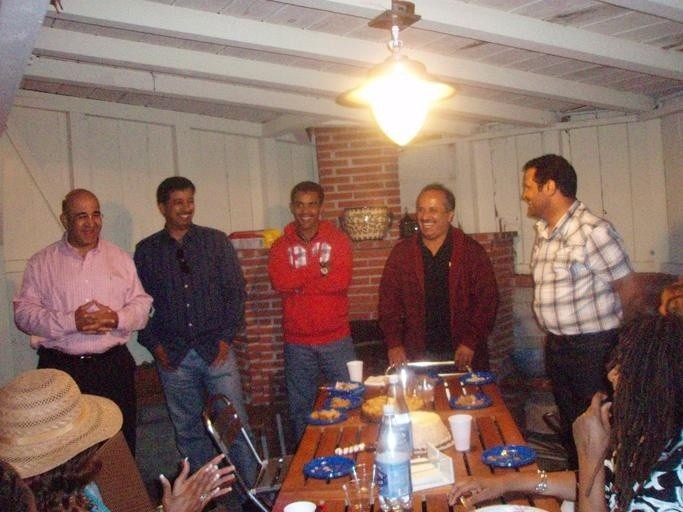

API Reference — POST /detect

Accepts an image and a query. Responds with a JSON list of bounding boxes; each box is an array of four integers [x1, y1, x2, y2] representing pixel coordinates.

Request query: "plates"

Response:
[[470, 501, 548, 512], [481, 444, 537, 468], [305, 457, 354, 478], [452, 392, 496, 409], [306, 380, 365, 424], [460, 370, 497, 384]]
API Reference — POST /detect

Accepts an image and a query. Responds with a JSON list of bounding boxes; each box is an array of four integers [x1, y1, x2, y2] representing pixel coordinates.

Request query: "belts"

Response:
[[43, 343, 125, 363]]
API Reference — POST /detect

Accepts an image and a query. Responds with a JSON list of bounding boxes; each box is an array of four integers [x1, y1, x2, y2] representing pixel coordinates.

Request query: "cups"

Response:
[[284, 500, 316, 511], [345, 359, 363, 383], [448, 413, 473, 452], [399, 361, 435, 408], [343, 463, 374, 511]]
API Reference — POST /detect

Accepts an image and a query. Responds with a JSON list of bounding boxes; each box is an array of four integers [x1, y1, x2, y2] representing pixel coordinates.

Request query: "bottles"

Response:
[[398, 207, 420, 238], [376, 377, 414, 512]]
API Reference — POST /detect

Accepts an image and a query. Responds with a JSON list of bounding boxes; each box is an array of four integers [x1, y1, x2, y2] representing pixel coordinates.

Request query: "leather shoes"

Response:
[[202, 496, 272, 512]]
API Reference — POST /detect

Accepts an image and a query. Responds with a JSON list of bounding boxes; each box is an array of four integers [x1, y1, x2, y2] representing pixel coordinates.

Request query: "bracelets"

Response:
[[534, 469, 549, 497]]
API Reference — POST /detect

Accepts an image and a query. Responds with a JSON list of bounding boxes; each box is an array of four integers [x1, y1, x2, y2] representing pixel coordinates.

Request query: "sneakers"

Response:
[[560, 499, 576, 512]]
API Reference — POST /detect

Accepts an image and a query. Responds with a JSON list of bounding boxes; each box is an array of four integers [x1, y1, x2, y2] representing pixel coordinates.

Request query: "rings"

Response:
[[197, 491, 212, 504]]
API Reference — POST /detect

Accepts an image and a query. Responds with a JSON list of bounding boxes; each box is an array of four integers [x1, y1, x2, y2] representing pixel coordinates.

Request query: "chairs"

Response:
[[85, 432, 156, 512], [200, 394, 294, 512]]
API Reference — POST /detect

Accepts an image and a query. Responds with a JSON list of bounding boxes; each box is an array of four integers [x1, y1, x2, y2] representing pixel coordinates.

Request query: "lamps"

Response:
[[334, 0, 457, 149]]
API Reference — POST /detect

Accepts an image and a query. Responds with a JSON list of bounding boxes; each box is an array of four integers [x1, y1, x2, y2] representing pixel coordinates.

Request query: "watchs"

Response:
[[319, 263, 328, 275]]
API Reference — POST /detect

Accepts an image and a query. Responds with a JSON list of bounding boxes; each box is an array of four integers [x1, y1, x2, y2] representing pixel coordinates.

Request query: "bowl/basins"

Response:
[[342, 205, 390, 240]]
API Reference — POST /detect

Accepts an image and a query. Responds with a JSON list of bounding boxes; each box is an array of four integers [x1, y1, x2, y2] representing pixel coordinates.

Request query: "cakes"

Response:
[[362, 395, 395, 419], [456, 394, 483, 405], [310, 407, 341, 419], [410, 411, 451, 448], [331, 397, 348, 409]]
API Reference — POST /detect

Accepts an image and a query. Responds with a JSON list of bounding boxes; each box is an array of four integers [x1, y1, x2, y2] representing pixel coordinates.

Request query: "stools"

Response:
[[247, 403, 287, 458]]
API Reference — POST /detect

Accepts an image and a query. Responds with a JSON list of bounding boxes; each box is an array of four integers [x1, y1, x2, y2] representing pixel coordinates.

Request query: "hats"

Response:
[[0, 368, 124, 480]]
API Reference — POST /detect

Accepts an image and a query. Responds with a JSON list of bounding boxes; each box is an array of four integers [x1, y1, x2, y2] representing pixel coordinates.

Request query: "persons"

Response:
[[447, 312, 683, 511], [0, 367, 238, 512], [521, 153, 650, 468], [267, 180, 363, 456], [14, 188, 153, 456], [127, 174, 260, 512], [377, 183, 498, 374]]
